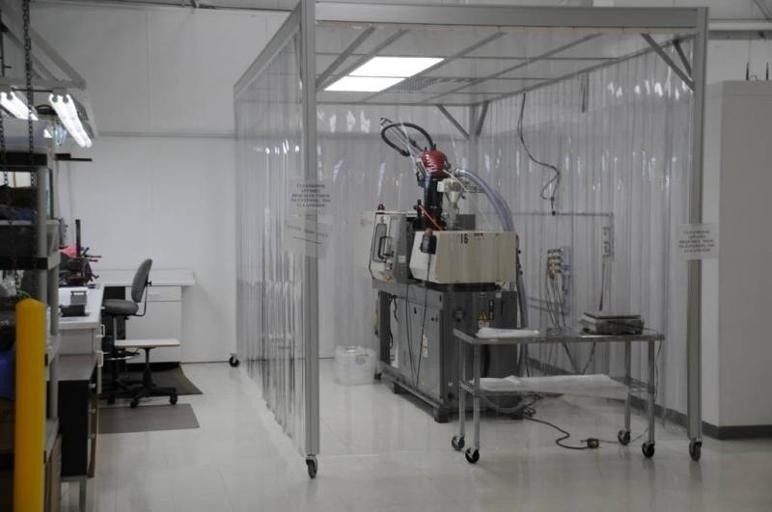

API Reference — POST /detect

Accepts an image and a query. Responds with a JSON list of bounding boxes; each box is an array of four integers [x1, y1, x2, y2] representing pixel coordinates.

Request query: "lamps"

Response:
[[0, 86, 39, 122], [48, 88, 92, 148]]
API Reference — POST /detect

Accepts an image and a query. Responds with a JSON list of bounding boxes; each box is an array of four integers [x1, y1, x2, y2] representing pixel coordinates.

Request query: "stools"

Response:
[[113, 339, 179, 407]]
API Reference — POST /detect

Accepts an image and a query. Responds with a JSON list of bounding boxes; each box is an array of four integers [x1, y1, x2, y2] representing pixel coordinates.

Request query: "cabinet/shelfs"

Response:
[[123, 286, 181, 362]]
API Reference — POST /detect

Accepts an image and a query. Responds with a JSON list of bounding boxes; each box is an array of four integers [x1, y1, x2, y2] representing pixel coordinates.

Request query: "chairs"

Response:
[[102, 259, 153, 396]]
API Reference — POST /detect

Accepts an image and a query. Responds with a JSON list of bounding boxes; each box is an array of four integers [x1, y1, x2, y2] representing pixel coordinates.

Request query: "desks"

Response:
[[40, 266, 194, 480]]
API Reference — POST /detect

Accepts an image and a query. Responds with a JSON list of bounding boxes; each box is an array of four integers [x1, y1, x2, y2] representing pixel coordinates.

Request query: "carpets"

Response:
[[90, 360, 201, 396]]
